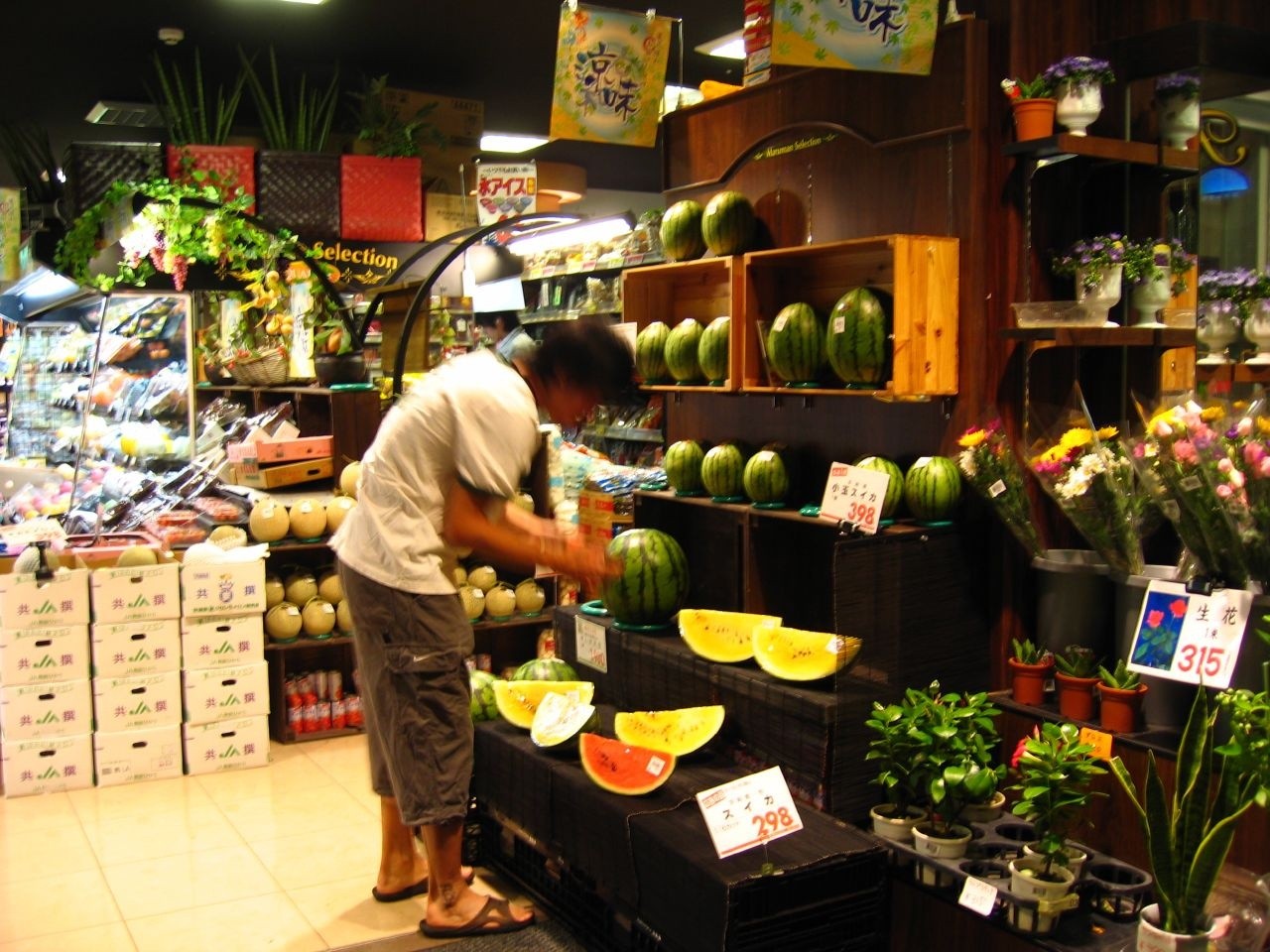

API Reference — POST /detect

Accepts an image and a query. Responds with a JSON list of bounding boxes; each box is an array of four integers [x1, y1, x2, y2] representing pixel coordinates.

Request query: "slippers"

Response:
[[419, 895, 535, 938], [371, 867, 476, 903]]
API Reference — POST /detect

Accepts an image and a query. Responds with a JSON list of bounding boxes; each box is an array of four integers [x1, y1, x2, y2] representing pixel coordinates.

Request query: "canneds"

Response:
[[284, 670, 364, 733]]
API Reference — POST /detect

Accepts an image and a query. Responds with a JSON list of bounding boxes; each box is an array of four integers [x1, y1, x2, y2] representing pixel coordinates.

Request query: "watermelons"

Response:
[[637, 316, 731, 380], [752, 624, 863, 681], [470, 657, 725, 795], [852, 452, 904, 520], [664, 439, 706, 492], [744, 449, 803, 503], [701, 444, 747, 498], [660, 191, 757, 261], [600, 528, 689, 624], [768, 285, 892, 385], [677, 610, 783, 663], [905, 455, 961, 515]]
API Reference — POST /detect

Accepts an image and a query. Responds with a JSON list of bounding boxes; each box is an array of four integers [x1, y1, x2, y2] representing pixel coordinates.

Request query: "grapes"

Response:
[[130, 228, 188, 292]]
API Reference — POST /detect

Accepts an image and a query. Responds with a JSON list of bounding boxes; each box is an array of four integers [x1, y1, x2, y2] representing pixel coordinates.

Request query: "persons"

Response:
[[475, 309, 536, 365], [327, 316, 648, 938]]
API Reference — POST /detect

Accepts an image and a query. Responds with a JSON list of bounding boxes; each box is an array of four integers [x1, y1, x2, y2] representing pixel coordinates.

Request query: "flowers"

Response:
[[958, 54, 1269, 598]]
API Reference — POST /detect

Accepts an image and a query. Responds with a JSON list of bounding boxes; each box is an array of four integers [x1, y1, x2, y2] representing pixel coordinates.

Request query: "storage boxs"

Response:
[[0, 434, 336, 799], [425, 192, 479, 245]]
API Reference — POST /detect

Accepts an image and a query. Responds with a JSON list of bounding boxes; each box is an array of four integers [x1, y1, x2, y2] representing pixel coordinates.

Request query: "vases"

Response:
[[1196, 301, 1241, 365], [1057, 78, 1104, 137], [1074, 262, 1125, 329], [59, 141, 164, 216], [1240, 298, 1270, 366], [1158, 96, 1201, 151], [1130, 265, 1178, 328]]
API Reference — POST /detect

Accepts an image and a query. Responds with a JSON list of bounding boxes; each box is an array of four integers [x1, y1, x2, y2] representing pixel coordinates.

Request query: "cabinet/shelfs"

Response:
[[619, 482, 990, 681], [518, 252, 668, 326], [1000, 132, 1200, 360], [868, 579, 1270, 952], [620, 235, 961, 404], [429, 296, 474, 367], [351, 278, 430, 382], [464, 429, 558, 674], [266, 538, 556, 743]]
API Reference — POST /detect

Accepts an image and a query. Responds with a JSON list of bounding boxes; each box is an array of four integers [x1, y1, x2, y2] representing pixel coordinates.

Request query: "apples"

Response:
[[141, 299, 185, 360], [255, 271, 294, 335], [19, 464, 127, 520]]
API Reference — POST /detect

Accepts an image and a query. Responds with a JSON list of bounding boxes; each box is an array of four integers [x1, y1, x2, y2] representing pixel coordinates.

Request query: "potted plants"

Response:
[[295, 261, 354, 386], [866, 636, 1270, 952], [192, 290, 242, 385], [147, 43, 424, 242], [1001, 75, 1056, 142], [0, 107, 66, 269]]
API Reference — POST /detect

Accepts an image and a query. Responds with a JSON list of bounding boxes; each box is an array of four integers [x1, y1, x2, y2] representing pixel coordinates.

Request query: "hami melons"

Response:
[[515, 491, 535, 513], [13, 545, 158, 574], [340, 460, 360, 500], [454, 547, 545, 619], [208, 495, 355, 639]]
